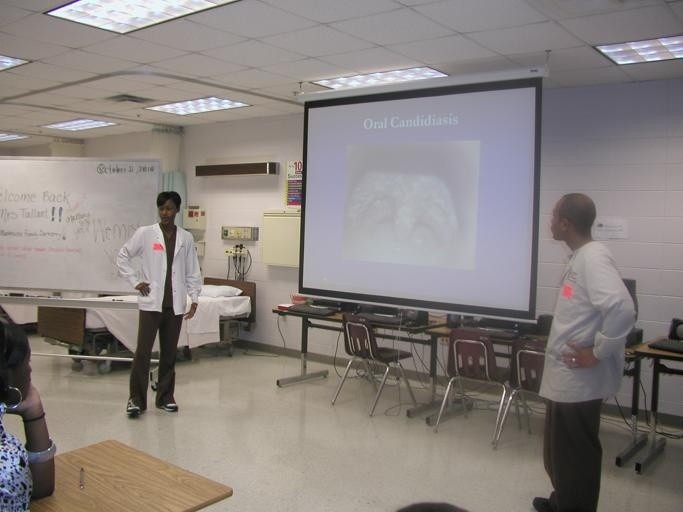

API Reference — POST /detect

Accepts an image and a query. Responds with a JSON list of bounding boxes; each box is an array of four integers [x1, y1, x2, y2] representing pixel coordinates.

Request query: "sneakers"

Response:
[[127, 398, 144, 415], [156, 400, 178, 413], [532, 496, 557, 511]]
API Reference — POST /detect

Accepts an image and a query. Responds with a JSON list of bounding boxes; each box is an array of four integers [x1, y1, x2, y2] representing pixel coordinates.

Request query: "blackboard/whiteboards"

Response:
[[0, 156, 164, 310]]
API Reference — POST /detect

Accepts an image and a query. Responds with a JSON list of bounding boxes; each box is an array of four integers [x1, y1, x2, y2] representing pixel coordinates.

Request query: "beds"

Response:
[[35, 274, 256, 373]]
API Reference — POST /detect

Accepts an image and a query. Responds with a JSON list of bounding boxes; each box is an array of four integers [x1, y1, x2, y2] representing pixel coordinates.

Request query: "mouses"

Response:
[[407, 321, 418, 327]]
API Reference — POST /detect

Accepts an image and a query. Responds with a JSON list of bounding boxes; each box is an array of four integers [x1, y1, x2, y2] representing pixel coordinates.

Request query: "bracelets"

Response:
[[22, 411, 47, 425], [25, 438, 56, 465]]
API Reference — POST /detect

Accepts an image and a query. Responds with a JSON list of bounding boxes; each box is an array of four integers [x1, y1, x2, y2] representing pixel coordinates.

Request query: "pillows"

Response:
[[196, 282, 245, 299]]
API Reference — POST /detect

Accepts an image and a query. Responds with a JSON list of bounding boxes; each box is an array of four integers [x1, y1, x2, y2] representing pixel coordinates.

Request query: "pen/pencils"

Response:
[[47, 296, 63, 298], [80, 467, 85, 490], [5, 293, 23, 296], [112, 299, 124, 301], [25, 295, 45, 297]]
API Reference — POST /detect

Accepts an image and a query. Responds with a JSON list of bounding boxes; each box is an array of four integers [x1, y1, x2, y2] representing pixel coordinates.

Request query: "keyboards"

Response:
[[480, 328, 519, 339], [357, 312, 403, 325], [287, 302, 334, 317], [648, 338, 683, 353]]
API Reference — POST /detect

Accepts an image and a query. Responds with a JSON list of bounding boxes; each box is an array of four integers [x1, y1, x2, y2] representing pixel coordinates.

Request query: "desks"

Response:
[[420, 318, 651, 468], [268, 300, 464, 419], [631, 334, 682, 473], [25, 437, 234, 512]]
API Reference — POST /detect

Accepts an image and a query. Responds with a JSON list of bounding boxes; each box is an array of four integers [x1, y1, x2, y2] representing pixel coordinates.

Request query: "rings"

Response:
[[571, 357, 576, 363]]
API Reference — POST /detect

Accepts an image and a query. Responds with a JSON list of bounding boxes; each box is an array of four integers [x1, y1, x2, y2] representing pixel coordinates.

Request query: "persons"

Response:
[[114, 190, 203, 417], [532, 192, 638, 512], [0, 324, 57, 512]]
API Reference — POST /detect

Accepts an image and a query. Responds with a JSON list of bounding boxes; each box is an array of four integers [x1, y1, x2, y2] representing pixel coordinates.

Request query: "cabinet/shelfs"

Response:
[[259, 209, 302, 270]]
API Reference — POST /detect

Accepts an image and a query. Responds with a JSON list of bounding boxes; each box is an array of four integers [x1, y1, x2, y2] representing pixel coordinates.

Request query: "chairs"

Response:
[[428, 327, 523, 447], [489, 336, 552, 450], [328, 311, 419, 418]]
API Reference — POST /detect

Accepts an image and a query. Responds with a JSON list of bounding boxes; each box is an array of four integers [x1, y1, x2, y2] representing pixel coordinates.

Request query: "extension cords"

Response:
[[225, 247, 249, 257]]
